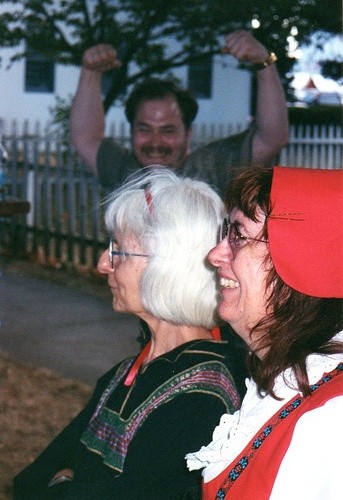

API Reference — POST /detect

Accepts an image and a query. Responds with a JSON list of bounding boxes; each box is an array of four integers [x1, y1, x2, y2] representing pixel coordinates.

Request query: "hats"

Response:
[[268, 164, 343, 302]]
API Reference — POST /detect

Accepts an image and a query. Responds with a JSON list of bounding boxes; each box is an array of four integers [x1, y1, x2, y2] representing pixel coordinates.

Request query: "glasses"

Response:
[[107, 237, 148, 268], [218, 217, 268, 248]]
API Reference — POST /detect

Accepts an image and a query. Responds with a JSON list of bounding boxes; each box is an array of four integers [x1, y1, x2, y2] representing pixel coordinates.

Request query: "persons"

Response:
[[69, 29, 290, 215], [185, 167, 343, 500], [11, 165, 250, 500]]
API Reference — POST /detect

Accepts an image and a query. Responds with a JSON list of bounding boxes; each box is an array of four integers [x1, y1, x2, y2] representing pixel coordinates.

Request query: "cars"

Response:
[[284, 72, 343, 110]]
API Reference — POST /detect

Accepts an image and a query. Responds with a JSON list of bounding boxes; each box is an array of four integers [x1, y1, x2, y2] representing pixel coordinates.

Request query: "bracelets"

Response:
[[249, 52, 277, 73], [49, 475, 72, 485]]
